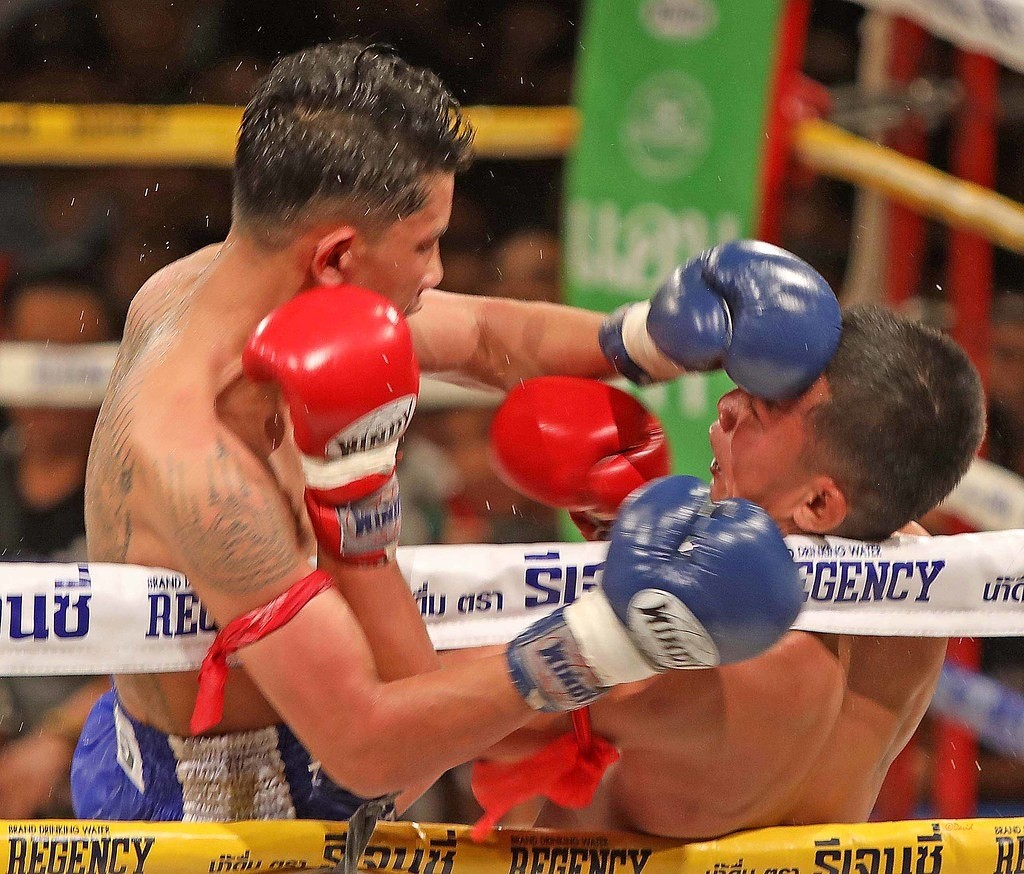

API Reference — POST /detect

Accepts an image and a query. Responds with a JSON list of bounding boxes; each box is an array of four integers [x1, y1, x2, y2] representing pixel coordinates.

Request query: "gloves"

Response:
[[489, 375, 670, 541], [507, 474, 803, 709], [243, 284, 419, 565], [599, 238, 842, 401]]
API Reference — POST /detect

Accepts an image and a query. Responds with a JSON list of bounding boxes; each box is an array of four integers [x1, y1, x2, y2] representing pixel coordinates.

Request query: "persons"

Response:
[[0, 1, 1024, 842]]
